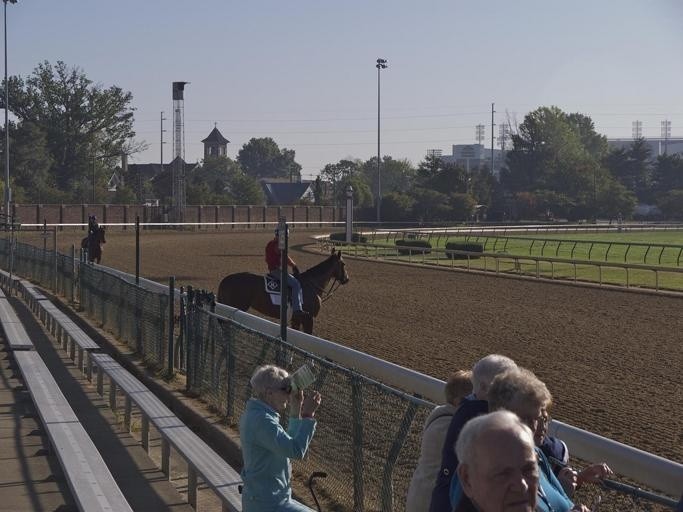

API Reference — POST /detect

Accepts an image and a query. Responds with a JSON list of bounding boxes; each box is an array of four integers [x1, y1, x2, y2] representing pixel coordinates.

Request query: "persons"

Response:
[[89, 216, 97, 233], [264, 223, 309, 317], [237, 364, 321, 512], [405, 353, 614, 512]]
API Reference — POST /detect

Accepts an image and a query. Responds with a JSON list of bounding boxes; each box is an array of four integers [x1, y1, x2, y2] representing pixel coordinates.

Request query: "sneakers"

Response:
[[293, 310, 310, 319]]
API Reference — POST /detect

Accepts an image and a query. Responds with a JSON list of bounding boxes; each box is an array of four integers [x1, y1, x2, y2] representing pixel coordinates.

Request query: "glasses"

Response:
[[279, 385, 292, 394]]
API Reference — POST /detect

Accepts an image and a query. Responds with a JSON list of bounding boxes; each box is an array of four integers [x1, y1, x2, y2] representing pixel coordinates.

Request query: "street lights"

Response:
[[345, 184, 353, 243], [375, 58, 387, 218], [3, 0, 18, 228]]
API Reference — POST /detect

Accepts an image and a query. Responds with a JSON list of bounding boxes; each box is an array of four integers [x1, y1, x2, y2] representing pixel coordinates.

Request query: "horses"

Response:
[[215, 247, 350, 335], [80, 225, 106, 264]]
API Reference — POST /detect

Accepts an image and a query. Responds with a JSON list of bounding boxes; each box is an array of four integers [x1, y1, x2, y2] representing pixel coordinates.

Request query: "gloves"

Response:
[[292, 265, 300, 274]]
